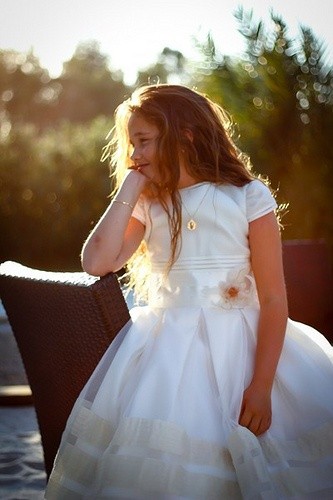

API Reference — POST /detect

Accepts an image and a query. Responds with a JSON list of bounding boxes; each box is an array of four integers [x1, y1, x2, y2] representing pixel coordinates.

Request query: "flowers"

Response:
[[217, 270, 256, 311]]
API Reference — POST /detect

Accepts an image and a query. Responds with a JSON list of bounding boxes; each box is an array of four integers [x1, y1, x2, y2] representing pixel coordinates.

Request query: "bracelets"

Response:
[[113, 199, 133, 210]]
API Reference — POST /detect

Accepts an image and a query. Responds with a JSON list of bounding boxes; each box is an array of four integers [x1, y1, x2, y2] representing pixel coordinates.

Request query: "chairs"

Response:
[[0, 261, 132, 487]]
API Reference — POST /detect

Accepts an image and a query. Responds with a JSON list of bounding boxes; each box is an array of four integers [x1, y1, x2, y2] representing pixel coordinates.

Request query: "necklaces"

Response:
[[167, 182, 212, 231]]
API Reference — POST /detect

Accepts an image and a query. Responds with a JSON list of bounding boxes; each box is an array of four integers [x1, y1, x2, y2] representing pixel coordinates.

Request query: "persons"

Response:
[[39, 82, 333, 499]]
